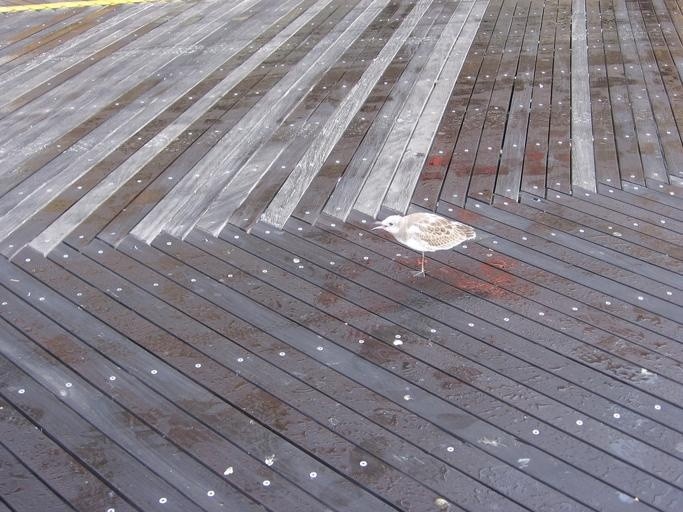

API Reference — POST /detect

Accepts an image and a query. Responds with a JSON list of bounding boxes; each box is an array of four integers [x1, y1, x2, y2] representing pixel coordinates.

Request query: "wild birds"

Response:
[[371, 212, 476, 278]]
